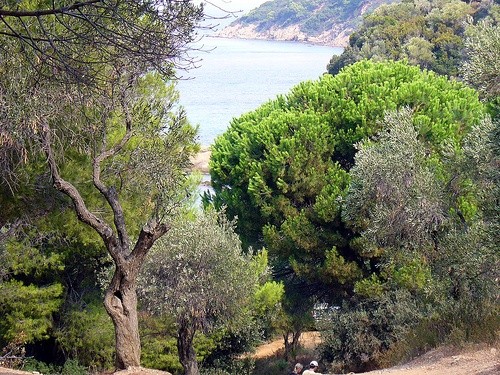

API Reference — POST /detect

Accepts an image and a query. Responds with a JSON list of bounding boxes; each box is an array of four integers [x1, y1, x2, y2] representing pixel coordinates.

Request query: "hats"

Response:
[[310, 361, 318, 366]]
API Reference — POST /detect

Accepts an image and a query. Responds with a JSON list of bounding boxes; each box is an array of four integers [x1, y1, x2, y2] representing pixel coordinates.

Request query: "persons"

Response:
[[302, 360, 323, 375], [290, 362, 303, 375]]
